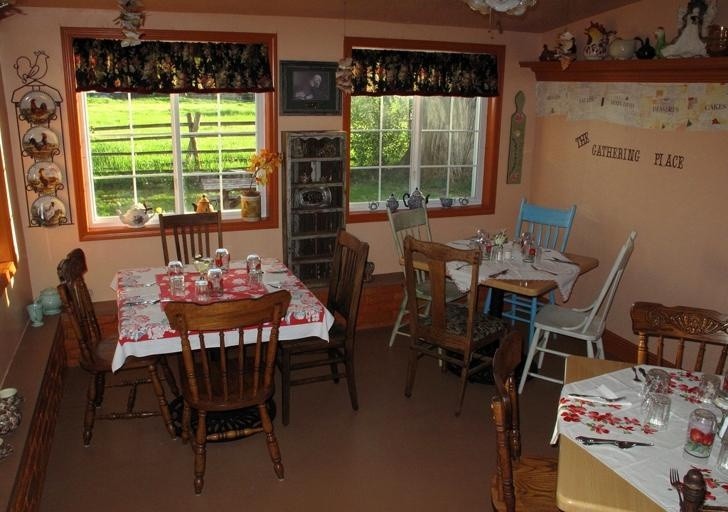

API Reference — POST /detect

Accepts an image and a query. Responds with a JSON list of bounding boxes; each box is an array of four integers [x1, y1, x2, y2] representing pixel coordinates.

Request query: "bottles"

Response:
[[386, 193, 399, 212], [33, 287, 63, 316], [0, 386, 25, 459]]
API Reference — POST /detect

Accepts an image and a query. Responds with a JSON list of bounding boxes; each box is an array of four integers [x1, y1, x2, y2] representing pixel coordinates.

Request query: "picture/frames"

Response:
[[276, 54, 343, 119]]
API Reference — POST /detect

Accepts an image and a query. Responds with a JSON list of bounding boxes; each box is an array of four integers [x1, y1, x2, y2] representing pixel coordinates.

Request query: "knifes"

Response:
[[574, 435, 653, 447]]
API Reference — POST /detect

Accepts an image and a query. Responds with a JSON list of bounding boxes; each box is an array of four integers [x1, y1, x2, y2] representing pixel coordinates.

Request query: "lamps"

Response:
[[467, 0, 537, 30]]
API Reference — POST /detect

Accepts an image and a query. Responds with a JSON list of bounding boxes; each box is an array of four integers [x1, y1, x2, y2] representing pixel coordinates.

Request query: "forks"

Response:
[[669, 467, 686, 508]]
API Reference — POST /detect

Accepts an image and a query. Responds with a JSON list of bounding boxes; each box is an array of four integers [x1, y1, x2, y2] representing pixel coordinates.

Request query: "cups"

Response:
[[640, 368, 671, 431], [311, 161, 321, 182], [168, 261, 184, 279], [171, 276, 184, 296], [697, 373, 720, 403], [195, 280, 208, 301], [246, 254, 262, 281], [468, 225, 537, 264], [27, 303, 45, 328], [439, 197, 456, 208]]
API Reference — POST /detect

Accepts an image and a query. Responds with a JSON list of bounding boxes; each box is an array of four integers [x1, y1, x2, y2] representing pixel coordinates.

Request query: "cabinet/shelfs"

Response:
[[0, 306, 71, 512], [281, 125, 350, 292]]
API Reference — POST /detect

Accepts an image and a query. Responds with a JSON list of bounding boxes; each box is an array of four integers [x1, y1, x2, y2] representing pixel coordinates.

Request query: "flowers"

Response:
[[241, 147, 282, 199]]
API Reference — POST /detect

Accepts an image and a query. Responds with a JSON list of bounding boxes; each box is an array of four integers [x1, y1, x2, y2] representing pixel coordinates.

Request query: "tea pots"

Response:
[[116, 202, 155, 229], [192, 195, 217, 213], [403, 187, 429, 210]]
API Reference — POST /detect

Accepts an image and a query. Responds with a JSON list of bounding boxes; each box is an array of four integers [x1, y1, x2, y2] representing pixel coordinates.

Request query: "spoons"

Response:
[[580, 440, 637, 449]]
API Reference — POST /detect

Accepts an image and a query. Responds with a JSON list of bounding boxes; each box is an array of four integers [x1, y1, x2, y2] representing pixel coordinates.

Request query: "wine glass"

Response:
[[191, 256, 213, 280]]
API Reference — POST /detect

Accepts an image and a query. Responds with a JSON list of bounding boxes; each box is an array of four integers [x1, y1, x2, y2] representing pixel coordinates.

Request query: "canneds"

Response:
[[480, 238, 491, 260], [522, 240, 536, 263], [168, 260, 183, 276], [246, 255, 261, 275], [477, 228, 489, 244], [521, 231, 532, 246]]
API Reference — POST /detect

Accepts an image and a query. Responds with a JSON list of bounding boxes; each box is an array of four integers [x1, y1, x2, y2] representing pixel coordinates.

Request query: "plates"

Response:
[[26, 162, 62, 196], [23, 126, 60, 159], [33, 198, 67, 226], [22, 91, 56, 124]]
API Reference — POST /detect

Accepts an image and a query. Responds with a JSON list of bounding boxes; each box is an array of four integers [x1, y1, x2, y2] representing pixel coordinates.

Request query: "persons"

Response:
[[294, 73, 328, 100], [660, 7, 707, 58]]
